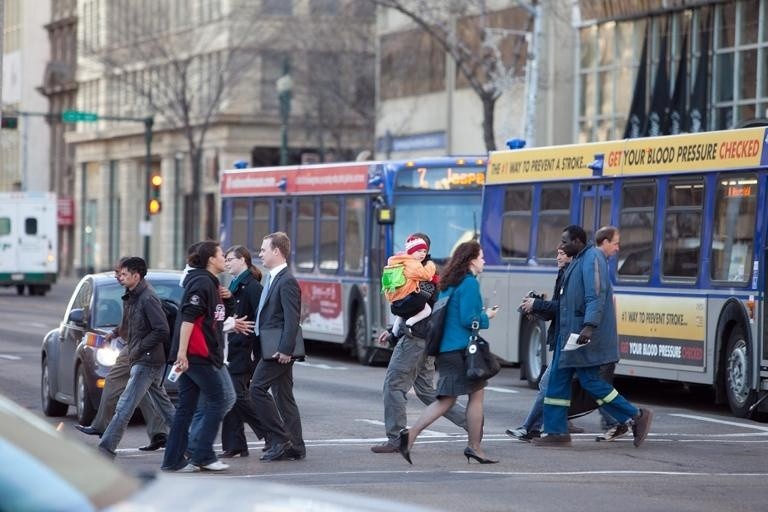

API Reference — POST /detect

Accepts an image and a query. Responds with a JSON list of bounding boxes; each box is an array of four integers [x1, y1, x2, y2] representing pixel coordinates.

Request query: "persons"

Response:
[[73, 257, 176, 462], [396, 241, 498, 465], [383, 236, 435, 348], [370, 232, 484, 452], [162, 233, 306, 471], [507, 225, 654, 447]]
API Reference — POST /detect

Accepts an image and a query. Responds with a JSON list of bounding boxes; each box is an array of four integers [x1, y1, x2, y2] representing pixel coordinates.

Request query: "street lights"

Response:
[[276, 73, 293, 166]]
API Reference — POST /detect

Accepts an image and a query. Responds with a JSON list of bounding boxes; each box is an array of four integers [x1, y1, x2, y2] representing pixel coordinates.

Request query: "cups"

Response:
[[167, 362, 185, 383]]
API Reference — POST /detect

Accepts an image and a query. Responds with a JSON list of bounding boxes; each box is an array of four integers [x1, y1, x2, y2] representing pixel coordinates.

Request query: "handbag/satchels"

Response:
[[465, 336, 500, 381], [261, 326, 304, 363], [423, 308, 446, 355]]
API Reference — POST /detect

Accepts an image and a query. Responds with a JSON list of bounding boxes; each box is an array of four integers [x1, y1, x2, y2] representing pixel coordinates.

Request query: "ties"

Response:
[[254, 274, 270, 335]]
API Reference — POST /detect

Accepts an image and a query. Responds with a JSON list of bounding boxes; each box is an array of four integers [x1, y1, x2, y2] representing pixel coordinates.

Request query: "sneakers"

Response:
[[371, 443, 401, 452], [176, 464, 199, 472], [505, 420, 583, 446], [260, 441, 306, 460], [203, 460, 229, 470], [386, 333, 397, 348], [400, 322, 414, 339], [598, 409, 653, 447]]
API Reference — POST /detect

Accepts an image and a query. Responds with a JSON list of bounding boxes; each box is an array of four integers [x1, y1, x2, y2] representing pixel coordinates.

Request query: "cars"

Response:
[[39, 268, 254, 426], [0, 389, 444, 512]]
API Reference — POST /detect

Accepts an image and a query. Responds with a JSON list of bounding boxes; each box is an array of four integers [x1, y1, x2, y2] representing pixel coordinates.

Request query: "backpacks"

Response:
[[160, 296, 180, 365], [380, 264, 406, 290]]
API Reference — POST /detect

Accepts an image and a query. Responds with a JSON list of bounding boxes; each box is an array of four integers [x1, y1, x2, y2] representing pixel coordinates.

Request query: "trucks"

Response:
[[0, 190, 58, 295]]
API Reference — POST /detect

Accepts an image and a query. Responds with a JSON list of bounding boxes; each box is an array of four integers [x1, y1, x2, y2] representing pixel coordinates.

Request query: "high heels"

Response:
[[395, 427, 412, 465], [464, 446, 498, 464]]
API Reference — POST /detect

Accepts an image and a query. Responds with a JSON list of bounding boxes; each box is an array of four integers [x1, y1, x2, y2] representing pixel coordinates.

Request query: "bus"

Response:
[[475, 124, 767, 420], [215, 154, 488, 364]]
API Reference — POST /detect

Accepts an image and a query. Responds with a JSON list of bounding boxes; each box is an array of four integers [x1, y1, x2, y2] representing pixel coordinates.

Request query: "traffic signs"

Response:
[[61, 110, 98, 123]]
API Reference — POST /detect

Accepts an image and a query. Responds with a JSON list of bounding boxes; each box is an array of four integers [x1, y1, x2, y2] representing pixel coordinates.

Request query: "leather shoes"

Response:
[[217, 447, 249, 457], [76, 424, 103, 437], [140, 434, 168, 452]]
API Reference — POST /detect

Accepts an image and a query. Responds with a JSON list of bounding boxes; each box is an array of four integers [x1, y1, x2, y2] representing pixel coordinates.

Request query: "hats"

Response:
[[406, 236, 428, 254]]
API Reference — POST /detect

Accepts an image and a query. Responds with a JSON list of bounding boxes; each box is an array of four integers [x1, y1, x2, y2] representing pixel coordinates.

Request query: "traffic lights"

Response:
[[149, 174, 162, 200], [149, 199, 160, 215], [1, 116, 18, 128]]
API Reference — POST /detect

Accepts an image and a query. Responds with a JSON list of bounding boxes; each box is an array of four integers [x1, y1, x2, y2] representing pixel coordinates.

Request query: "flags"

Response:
[[622, 13, 714, 139]]
